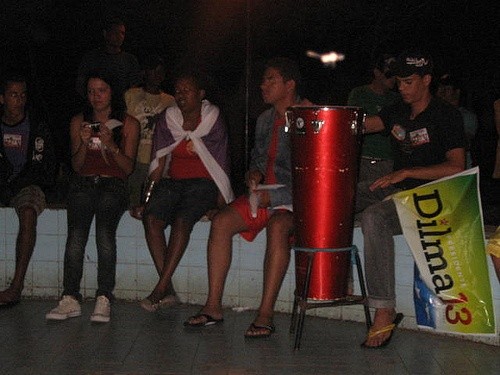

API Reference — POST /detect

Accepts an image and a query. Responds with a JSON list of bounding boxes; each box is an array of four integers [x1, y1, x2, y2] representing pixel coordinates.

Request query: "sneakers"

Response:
[[90, 294, 111, 322], [46, 295, 82, 320]]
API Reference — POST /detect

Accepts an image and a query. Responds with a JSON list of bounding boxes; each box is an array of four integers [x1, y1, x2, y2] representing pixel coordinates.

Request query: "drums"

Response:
[[283, 105, 368, 300]]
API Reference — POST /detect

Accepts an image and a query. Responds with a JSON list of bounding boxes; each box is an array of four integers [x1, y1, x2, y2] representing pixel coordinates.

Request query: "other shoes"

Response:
[[0, 289, 22, 305], [140, 285, 180, 312]]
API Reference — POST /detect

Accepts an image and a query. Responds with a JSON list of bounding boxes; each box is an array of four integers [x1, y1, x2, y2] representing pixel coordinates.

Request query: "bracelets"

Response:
[[112, 147, 120, 159]]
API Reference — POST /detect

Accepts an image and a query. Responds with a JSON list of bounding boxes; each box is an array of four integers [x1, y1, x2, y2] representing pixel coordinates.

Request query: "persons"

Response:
[[131, 70, 228, 315], [487, 225, 500, 282], [45, 69, 141, 323], [184, 56, 315, 339], [0, 72, 55, 309], [360, 49, 466, 348], [74, 18, 500, 214]]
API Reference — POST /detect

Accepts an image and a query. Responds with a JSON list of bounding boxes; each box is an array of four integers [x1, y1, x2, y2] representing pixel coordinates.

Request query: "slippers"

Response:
[[184, 313, 224, 328], [360, 313, 404, 348], [244, 323, 276, 337]]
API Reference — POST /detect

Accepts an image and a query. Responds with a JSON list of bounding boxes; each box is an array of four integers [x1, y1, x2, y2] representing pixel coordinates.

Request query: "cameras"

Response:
[[84, 120, 101, 134]]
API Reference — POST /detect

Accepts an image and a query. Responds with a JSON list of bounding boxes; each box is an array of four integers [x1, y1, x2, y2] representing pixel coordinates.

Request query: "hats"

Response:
[[439, 74, 463, 85], [384, 49, 433, 78]]
